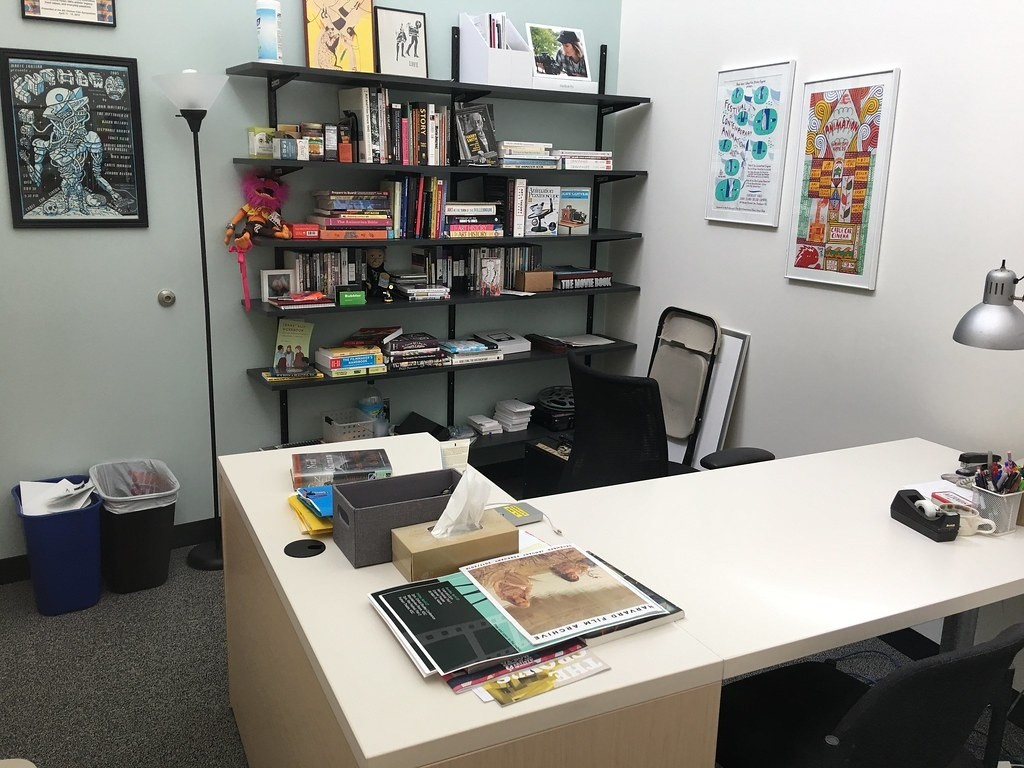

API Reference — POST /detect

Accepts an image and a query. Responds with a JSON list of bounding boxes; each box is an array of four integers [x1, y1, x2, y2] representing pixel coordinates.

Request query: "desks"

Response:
[[218, 432, 723, 768], [517, 439, 1024, 679]]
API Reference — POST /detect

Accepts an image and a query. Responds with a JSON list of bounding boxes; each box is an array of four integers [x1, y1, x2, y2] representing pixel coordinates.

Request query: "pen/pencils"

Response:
[[974, 451, 1024, 495]]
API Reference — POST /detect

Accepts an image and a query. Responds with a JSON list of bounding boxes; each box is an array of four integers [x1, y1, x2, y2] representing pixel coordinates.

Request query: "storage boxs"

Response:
[[320, 406, 375, 441], [972, 485, 1024, 535], [515, 270, 554, 291], [459, 13, 537, 88]]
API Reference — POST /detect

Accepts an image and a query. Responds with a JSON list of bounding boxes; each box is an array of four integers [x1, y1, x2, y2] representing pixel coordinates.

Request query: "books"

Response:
[[444, 175, 591, 238], [389, 244, 542, 301], [308, 172, 447, 240], [472, 13, 507, 50], [263, 318, 531, 382], [372, 544, 684, 707], [337, 87, 451, 166], [269, 246, 368, 310], [495, 140, 614, 171], [543, 266, 613, 290], [288, 448, 392, 490], [455, 102, 499, 167]]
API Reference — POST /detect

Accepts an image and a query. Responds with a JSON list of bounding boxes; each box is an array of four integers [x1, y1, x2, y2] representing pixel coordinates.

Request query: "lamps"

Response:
[[158, 67, 229, 569], [953, 261, 1024, 351]]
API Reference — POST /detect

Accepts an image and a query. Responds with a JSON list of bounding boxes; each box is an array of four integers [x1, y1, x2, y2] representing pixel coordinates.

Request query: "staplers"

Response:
[[956, 451, 1002, 477]]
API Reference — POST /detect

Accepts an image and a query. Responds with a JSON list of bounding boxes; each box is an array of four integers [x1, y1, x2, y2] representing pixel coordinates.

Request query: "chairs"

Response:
[[567, 349, 775, 491], [648, 306, 721, 476], [718, 621, 1024, 768]]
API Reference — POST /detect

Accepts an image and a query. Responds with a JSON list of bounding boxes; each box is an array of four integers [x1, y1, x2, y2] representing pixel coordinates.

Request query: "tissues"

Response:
[[391, 463, 519, 584]]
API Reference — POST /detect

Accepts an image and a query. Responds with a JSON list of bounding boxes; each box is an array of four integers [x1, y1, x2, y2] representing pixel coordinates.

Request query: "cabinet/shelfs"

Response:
[[226, 62, 651, 444]]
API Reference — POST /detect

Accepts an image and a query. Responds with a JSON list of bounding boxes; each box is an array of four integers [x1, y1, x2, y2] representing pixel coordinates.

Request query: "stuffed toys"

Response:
[[225, 169, 292, 251]]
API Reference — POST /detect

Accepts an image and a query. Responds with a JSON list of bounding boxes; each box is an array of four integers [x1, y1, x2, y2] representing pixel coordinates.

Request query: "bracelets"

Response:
[[225, 223, 235, 229]]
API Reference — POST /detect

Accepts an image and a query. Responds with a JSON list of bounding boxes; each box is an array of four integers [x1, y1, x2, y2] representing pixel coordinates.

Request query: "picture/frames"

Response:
[[705, 59, 796, 227], [303, 0, 376, 73], [525, 23, 591, 80], [374, 6, 429, 78], [785, 67, 900, 290], [21, 0, 116, 27], [0, 48, 150, 228], [668, 326, 751, 472]]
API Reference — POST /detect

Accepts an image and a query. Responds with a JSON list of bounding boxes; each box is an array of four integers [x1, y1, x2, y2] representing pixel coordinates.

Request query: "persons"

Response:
[[364, 246, 394, 303], [269, 276, 290, 296], [466, 113, 495, 155], [537, 31, 587, 77]]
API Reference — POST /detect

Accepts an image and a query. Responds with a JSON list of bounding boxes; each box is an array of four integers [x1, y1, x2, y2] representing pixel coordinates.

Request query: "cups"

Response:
[[939, 503, 996, 536]]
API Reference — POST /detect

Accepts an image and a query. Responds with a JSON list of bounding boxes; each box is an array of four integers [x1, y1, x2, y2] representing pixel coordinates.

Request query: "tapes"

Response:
[[914, 500, 937, 518]]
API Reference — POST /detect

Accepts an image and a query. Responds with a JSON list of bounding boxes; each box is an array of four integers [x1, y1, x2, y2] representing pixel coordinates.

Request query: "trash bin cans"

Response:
[[88, 459, 179, 595], [12, 474, 103, 617]]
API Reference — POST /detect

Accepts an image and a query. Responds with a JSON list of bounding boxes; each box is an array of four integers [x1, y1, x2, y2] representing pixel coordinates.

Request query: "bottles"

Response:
[[358, 380, 383, 422], [255, 0, 284, 64]]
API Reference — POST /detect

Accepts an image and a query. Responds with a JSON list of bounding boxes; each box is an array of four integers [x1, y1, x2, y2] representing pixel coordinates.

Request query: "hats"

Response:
[[555, 30, 580, 44]]
